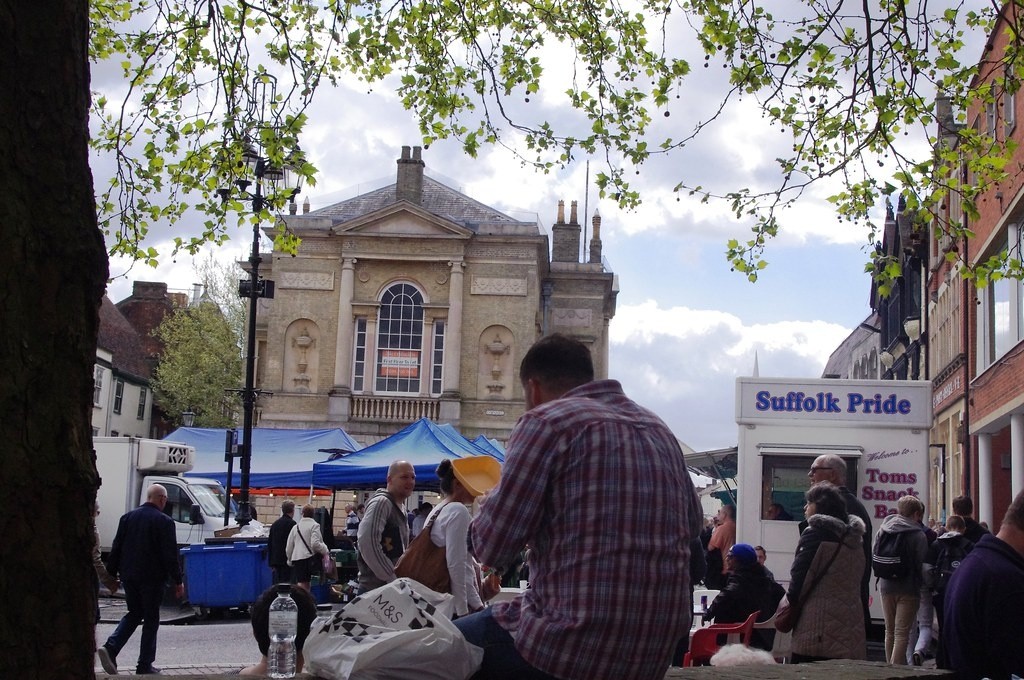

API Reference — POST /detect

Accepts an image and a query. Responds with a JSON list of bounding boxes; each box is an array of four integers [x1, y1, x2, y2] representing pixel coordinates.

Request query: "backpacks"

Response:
[[933, 540, 974, 597], [872, 528, 921, 581]]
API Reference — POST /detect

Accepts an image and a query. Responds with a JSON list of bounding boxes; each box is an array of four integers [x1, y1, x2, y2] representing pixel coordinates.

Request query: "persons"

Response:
[[93, 502, 122, 652], [943, 488, 1024, 680], [357, 460, 417, 597], [669, 503, 794, 667], [96, 484, 185, 675], [452, 333, 703, 680], [267, 501, 432, 604], [797, 454, 872, 637], [788, 480, 866, 663], [424, 460, 484, 618], [221, 582, 316, 674], [875, 495, 994, 668]]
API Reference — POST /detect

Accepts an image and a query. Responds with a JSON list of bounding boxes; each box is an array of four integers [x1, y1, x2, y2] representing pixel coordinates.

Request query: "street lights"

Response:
[[219, 69, 306, 526]]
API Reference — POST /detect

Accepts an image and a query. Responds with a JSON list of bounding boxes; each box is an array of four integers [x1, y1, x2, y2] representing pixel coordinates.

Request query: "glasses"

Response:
[[727, 552, 736, 560], [810, 467, 833, 475]]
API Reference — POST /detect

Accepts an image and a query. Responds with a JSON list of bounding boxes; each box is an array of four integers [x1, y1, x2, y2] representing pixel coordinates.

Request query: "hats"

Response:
[[729, 543, 757, 566]]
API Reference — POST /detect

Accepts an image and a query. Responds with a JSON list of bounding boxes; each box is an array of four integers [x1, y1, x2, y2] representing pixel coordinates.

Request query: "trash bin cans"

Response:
[[180, 538, 274, 619]]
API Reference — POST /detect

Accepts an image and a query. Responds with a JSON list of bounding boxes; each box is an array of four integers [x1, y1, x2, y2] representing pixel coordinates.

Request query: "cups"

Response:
[[701, 596, 707, 610], [520, 580, 530, 590]]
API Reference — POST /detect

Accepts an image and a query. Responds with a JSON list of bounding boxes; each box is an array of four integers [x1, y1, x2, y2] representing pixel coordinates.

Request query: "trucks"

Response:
[[93, 433, 271, 597], [735, 376, 932, 626]]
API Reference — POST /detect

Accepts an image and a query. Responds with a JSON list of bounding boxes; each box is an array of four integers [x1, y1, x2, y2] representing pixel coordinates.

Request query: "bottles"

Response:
[[310, 605, 333, 632], [268, 584, 298, 679]]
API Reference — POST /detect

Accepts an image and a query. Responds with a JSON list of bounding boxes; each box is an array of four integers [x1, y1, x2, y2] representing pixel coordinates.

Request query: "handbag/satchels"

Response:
[[301, 577, 484, 680], [324, 558, 339, 581], [774, 592, 805, 633], [311, 555, 322, 575], [392, 500, 452, 595]]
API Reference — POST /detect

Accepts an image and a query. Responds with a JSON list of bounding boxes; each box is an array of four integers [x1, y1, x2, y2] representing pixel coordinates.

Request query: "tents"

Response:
[[162, 415, 508, 531]]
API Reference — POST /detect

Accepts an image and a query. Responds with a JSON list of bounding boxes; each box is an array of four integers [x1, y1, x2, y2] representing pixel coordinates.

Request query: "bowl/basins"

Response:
[[452, 456, 504, 496]]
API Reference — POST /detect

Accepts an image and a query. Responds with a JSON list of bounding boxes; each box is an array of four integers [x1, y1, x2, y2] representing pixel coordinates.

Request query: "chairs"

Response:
[[727, 593, 792, 665], [683, 611, 759, 667]]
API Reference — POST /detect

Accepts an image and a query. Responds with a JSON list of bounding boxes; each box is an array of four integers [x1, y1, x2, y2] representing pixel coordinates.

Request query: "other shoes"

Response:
[[912, 651, 924, 666], [98, 646, 118, 675], [137, 666, 162, 675]]
